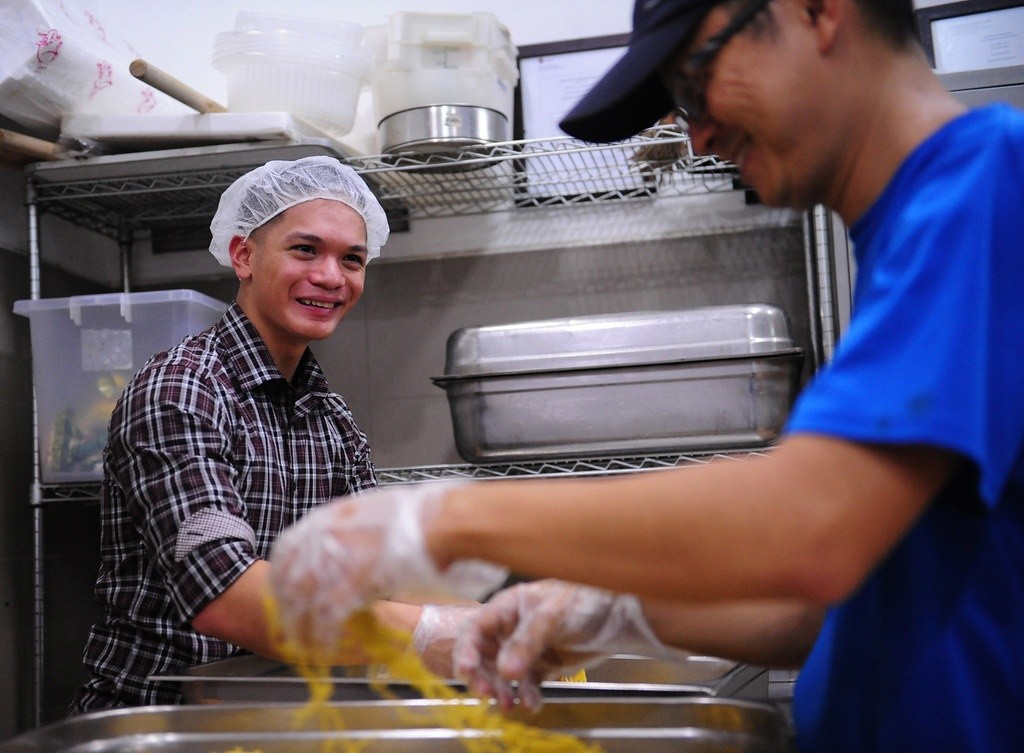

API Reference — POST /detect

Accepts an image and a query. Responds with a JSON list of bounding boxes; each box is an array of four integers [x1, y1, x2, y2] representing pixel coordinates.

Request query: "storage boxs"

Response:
[[12, 289, 231, 483]]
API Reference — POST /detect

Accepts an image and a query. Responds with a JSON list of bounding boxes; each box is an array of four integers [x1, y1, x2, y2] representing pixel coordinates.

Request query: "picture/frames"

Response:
[[913, 0, 1024, 72], [513, 31, 746, 207]]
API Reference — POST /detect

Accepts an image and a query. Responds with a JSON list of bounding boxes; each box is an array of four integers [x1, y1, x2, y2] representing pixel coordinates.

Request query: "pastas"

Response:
[[259, 584, 604, 753]]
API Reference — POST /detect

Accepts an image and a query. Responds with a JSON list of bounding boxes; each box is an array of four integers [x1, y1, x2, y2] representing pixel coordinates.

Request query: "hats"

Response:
[[559, 0, 713, 144]]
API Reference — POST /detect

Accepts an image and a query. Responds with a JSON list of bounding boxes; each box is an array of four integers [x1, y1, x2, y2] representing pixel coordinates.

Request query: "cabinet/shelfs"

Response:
[[8, 64, 1024, 727]]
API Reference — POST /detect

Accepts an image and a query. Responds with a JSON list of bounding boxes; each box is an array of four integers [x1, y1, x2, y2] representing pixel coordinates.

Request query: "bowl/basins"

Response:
[[378, 104, 508, 174], [212, 1, 386, 135]]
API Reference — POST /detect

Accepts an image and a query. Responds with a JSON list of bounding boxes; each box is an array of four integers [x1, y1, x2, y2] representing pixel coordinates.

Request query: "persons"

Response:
[[271, 0, 1024, 753], [64, 157, 483, 720]]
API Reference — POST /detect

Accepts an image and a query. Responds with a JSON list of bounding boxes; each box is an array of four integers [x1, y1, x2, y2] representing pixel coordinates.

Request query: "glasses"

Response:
[[666, 0, 766, 121]]
